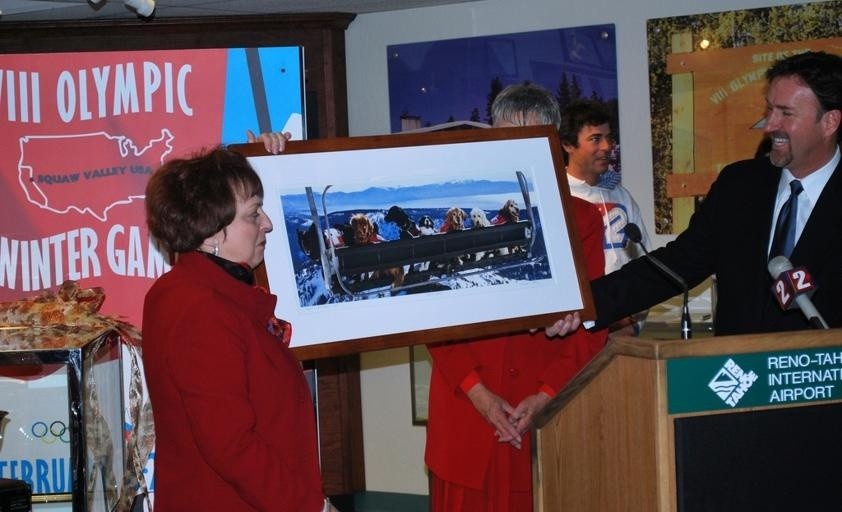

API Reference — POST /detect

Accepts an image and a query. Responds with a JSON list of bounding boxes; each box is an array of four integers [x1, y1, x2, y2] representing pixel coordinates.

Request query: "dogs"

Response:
[[469, 207, 509, 261], [323, 228, 344, 276], [439, 207, 471, 266], [418, 215, 438, 272], [385, 205, 421, 275], [349, 212, 405, 289], [491, 199, 523, 255]]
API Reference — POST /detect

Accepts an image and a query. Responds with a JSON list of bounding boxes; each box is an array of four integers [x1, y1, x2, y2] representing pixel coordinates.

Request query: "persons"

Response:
[[142, 131, 338, 512], [559, 107, 651, 275], [423, 85, 609, 512], [531, 52, 842, 340]]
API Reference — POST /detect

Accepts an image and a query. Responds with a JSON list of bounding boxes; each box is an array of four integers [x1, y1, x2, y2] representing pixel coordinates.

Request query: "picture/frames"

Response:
[[227, 120, 605, 370]]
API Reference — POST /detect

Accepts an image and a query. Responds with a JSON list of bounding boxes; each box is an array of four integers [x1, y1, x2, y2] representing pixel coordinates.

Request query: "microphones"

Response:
[[768, 256, 830, 330], [624, 223, 692, 334]]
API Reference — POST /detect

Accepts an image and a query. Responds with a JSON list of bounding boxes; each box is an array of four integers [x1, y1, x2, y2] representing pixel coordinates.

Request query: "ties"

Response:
[[767, 180, 803, 263]]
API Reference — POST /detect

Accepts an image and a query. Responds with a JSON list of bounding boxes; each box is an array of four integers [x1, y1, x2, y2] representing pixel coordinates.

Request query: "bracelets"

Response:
[[323, 497, 330, 512]]
